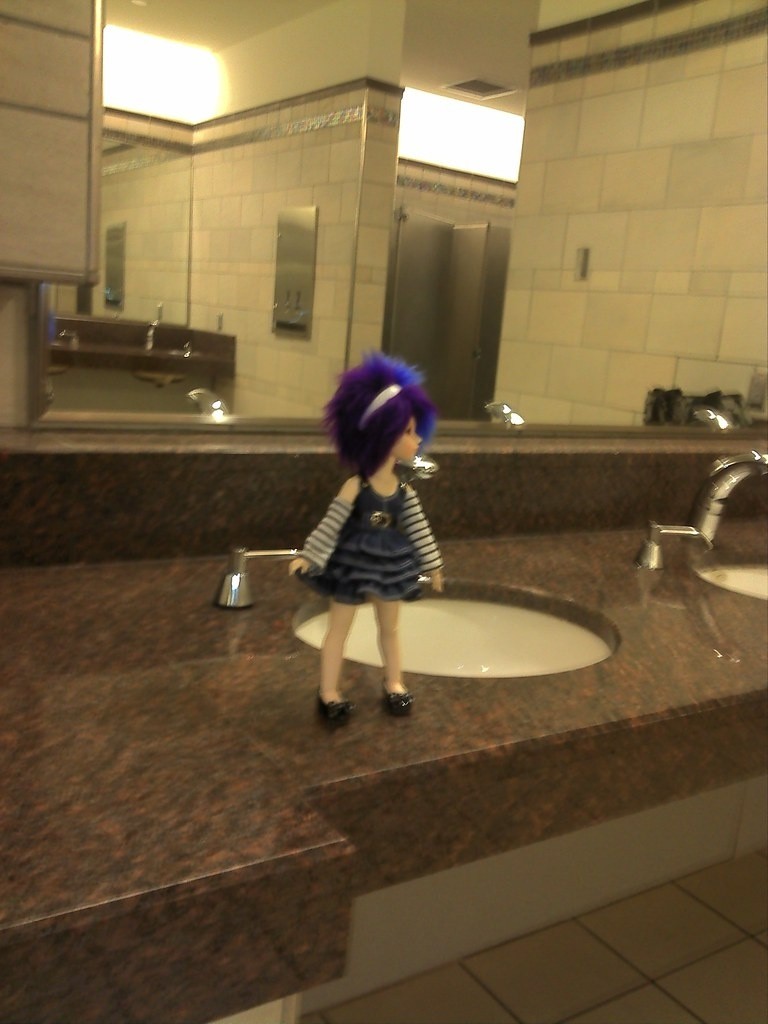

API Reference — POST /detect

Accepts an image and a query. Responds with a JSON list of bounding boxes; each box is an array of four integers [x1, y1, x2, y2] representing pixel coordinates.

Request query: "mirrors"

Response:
[[105, 222, 126, 308], [270, 206, 318, 341], [31, 0, 768, 439]]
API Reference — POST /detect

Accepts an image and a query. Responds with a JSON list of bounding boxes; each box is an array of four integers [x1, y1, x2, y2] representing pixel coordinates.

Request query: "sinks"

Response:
[[289, 573, 622, 680], [692, 559, 767, 605]]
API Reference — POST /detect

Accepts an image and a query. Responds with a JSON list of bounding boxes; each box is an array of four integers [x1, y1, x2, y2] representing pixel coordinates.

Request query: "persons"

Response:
[[288, 350, 445, 727]]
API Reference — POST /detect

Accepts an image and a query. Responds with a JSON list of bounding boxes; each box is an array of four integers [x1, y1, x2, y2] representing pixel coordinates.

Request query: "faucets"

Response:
[[687, 449, 767, 545], [183, 386, 229, 415], [676, 583, 751, 666], [391, 457, 440, 478], [483, 399, 524, 424]]
